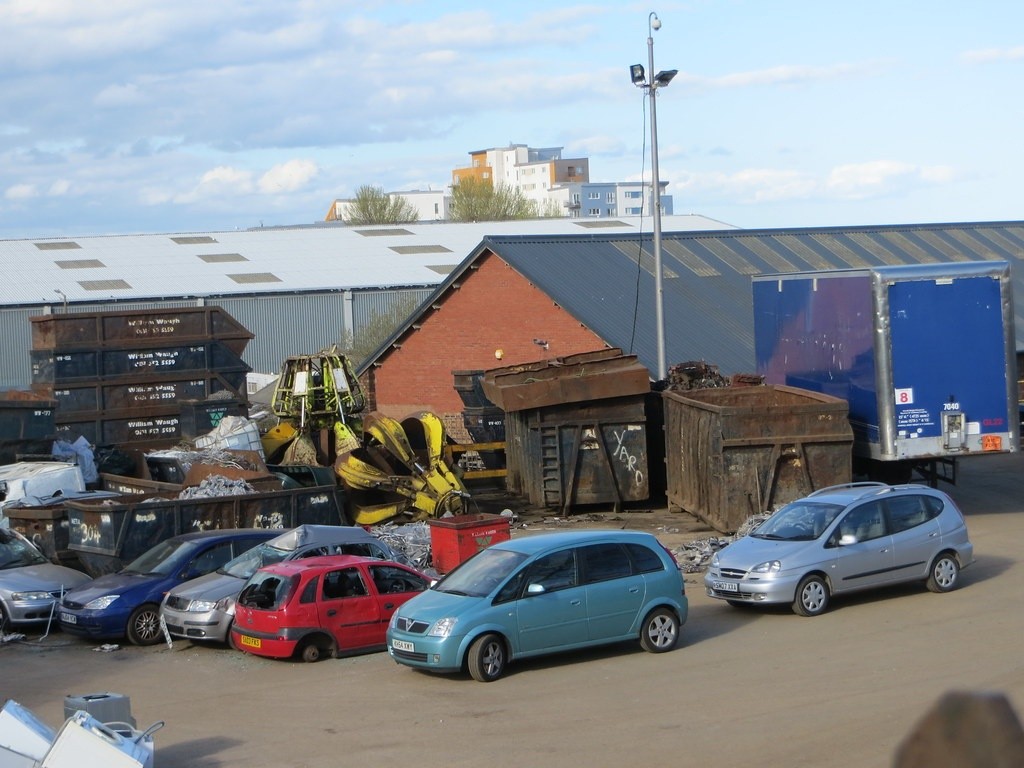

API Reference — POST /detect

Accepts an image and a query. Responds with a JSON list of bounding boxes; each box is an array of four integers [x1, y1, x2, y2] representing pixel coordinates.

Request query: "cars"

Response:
[[231, 553, 441, 664], [53, 527, 299, 647], [0, 526, 94, 636], [156, 523, 422, 651], [703, 481, 973, 617], [385, 528, 689, 682]]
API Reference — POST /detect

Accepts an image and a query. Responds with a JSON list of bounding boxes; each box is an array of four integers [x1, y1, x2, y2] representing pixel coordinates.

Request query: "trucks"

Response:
[[750, 261, 1022, 486]]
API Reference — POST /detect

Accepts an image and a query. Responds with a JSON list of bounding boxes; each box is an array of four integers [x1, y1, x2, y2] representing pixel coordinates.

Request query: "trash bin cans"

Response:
[[425, 514, 511, 574]]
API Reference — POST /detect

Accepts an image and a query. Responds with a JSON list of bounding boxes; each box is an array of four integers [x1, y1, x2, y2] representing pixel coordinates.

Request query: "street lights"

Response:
[[629, 11, 679, 382]]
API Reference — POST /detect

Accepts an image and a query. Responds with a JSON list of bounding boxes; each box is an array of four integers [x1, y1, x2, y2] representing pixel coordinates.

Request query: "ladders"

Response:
[[537, 425, 564, 511]]
